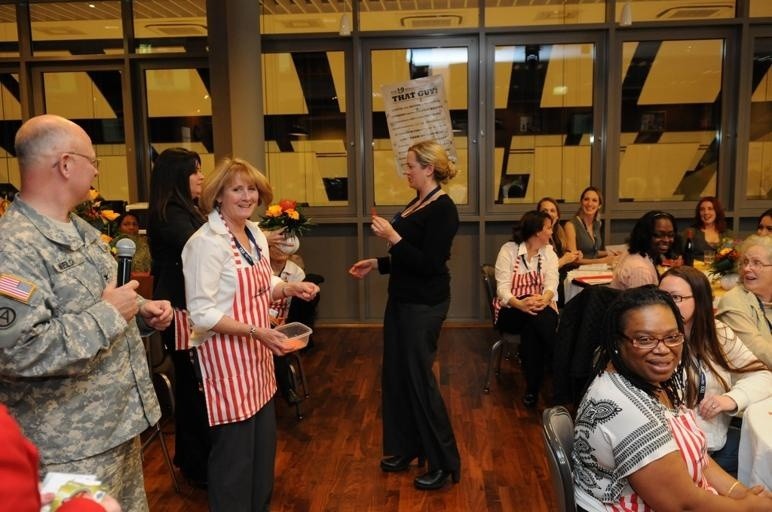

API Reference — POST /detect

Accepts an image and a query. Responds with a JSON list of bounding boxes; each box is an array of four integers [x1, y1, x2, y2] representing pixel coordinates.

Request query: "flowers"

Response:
[[73, 190, 120, 255], [258, 199, 312, 237], [715, 238, 740, 262]]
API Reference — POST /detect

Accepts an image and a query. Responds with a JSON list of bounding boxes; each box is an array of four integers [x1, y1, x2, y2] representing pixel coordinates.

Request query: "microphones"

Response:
[[115, 238, 137, 287]]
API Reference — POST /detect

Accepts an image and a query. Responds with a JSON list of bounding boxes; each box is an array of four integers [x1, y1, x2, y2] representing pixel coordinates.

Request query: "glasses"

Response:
[[673, 295, 694, 302], [621, 332, 684, 349]]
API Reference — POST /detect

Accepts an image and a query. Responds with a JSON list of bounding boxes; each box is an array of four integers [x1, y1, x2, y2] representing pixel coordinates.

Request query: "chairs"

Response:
[[271, 274, 323, 419], [542, 405, 577, 512], [140, 329, 181, 493], [481, 263, 521, 393]]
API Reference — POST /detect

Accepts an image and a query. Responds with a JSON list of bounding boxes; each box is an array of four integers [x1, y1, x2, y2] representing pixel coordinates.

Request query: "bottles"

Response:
[[683, 230, 696, 268]]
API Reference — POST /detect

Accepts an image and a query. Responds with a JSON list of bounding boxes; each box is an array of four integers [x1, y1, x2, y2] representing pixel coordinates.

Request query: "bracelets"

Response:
[[249, 328, 256, 337], [726, 481, 740, 496], [282, 287, 288, 298]]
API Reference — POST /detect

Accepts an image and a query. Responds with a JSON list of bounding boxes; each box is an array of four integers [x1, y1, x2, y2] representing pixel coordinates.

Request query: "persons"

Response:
[[495, 188, 772, 511], [181, 155, 321, 512], [348, 140, 460, 492], [1, 114, 175, 511], [0, 404, 121, 511], [117, 148, 306, 467]]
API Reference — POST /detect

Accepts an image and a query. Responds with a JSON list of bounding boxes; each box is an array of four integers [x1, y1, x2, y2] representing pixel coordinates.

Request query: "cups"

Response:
[[703, 250, 716, 265]]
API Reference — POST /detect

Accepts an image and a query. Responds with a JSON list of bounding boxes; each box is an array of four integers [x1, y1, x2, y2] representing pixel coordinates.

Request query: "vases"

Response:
[[275, 233, 300, 255], [714, 259, 734, 271]]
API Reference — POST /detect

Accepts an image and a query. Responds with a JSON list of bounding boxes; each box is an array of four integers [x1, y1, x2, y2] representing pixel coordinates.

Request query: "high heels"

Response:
[[382, 453, 425, 470], [415, 464, 459, 490]]
